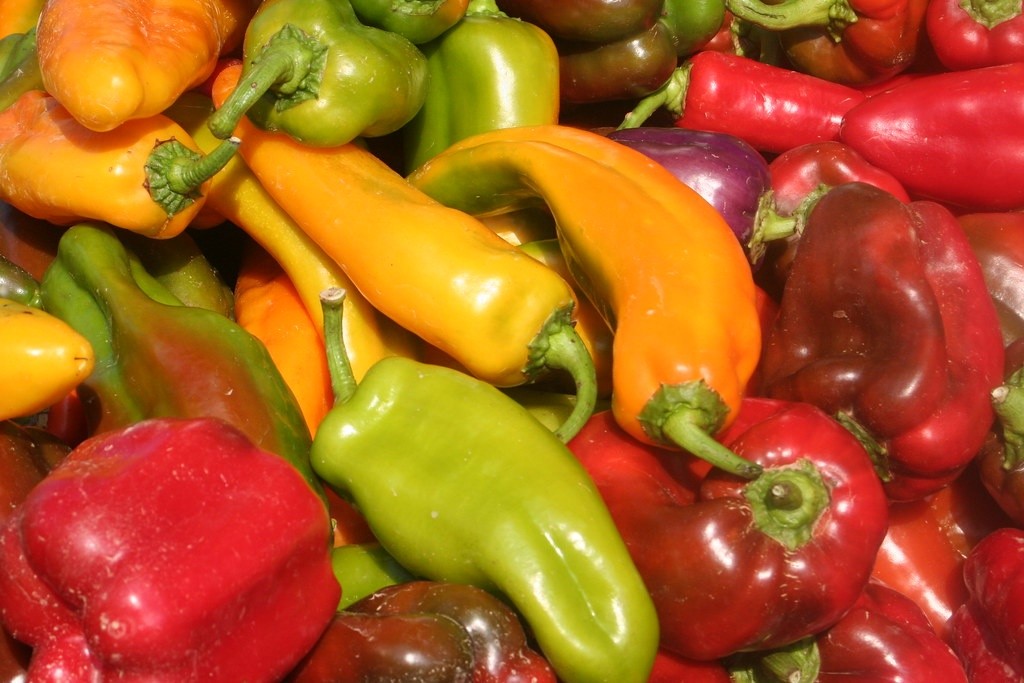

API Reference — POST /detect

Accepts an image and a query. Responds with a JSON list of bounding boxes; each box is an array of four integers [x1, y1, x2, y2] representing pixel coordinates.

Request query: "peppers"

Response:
[[0, 1, 1024, 683]]
